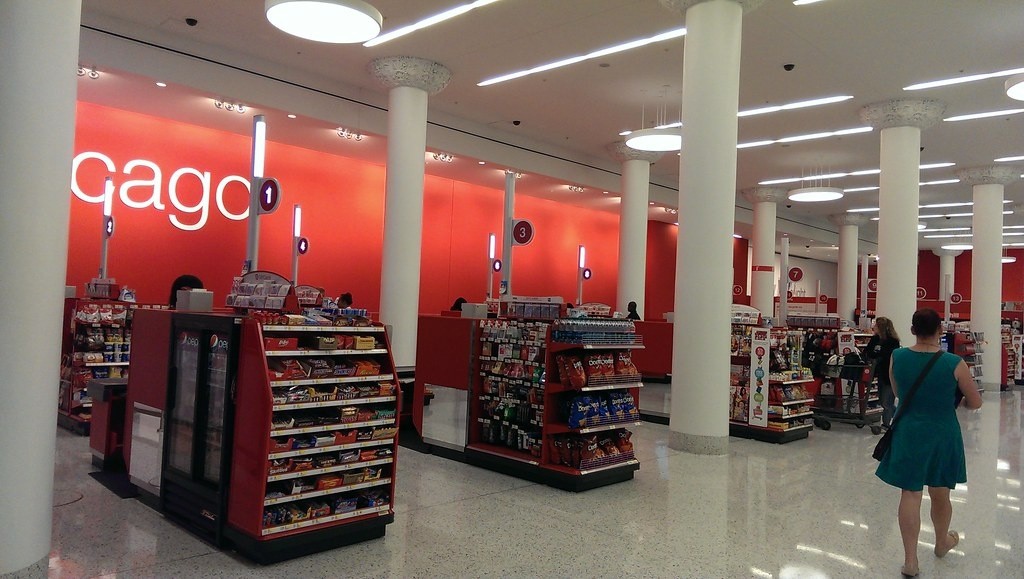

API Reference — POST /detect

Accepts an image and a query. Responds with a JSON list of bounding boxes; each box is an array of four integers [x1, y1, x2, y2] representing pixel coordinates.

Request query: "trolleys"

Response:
[[813, 355, 885, 436]]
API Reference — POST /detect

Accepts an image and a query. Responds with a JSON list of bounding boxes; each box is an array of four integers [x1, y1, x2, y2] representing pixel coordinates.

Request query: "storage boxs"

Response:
[[265, 336, 399, 525]]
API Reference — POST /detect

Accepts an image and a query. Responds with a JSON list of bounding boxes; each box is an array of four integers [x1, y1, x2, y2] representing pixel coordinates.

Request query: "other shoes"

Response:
[[901, 565, 919, 577], [934, 529, 960, 557]]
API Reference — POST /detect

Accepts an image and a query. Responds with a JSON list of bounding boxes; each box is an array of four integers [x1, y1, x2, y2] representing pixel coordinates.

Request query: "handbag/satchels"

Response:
[[873, 427, 894, 462]]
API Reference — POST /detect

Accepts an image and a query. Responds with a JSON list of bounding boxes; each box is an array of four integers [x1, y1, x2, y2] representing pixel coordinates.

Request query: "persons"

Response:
[[450, 298, 467, 311], [316, 287, 326, 298], [861, 316, 900, 434], [626, 301, 641, 320], [876, 309, 981, 578], [337, 292, 353, 308], [168, 275, 203, 310], [565, 303, 574, 317]]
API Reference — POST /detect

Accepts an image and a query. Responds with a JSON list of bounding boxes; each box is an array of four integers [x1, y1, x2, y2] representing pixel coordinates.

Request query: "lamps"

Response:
[[103, 176, 113, 216], [578, 244, 585, 268], [1002, 246, 1017, 264], [250, 113, 266, 179], [263, 1, 383, 43], [293, 203, 301, 238], [940, 230, 973, 251], [918, 220, 928, 230], [787, 165, 844, 202], [1005, 73, 1024, 101], [489, 232, 495, 259], [624, 102, 682, 152]]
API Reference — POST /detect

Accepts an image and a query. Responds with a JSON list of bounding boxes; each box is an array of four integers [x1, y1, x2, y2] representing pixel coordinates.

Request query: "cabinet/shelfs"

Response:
[[953, 332, 985, 394], [221, 316, 401, 566], [538, 326, 647, 494], [60, 297, 170, 437], [851, 333, 884, 416], [769, 378, 817, 445]]
